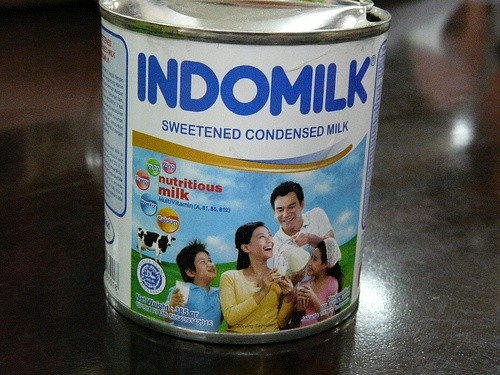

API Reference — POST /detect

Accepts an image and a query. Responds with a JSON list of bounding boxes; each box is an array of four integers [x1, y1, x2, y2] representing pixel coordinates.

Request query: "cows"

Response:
[[137, 227, 176, 263]]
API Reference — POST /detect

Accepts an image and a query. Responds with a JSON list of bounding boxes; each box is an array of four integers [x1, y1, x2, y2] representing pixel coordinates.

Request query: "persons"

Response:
[[293, 238, 343, 328], [163, 238, 224, 332], [266, 181, 336, 287], [219, 221, 294, 333]]
